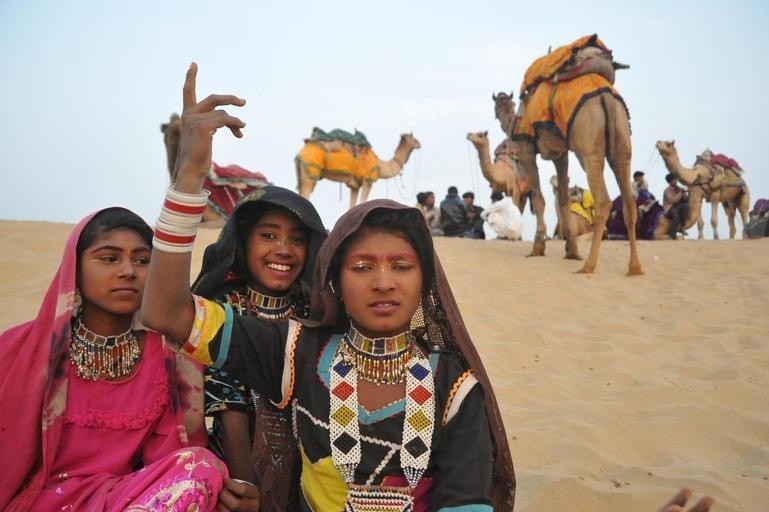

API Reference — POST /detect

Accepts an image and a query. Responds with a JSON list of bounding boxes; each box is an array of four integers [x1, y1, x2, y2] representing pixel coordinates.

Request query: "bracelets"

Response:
[[150, 180, 211, 256]]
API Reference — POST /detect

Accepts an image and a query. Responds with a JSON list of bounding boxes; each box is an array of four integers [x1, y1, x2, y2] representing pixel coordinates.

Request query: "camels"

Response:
[[294, 126, 421, 206], [468, 129, 528, 220], [655, 139, 749, 243], [490, 72, 642, 278], [601, 173, 722, 241], [549, 174, 597, 240]]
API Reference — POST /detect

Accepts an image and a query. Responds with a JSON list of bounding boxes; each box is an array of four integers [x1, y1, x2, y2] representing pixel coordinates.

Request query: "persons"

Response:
[[633, 171, 648, 191], [440, 185, 472, 236], [478, 191, 523, 241], [661, 171, 690, 240], [462, 191, 487, 239], [139, 59, 517, 512], [188, 184, 329, 511], [1, 206, 231, 512], [412, 191, 444, 236]]
